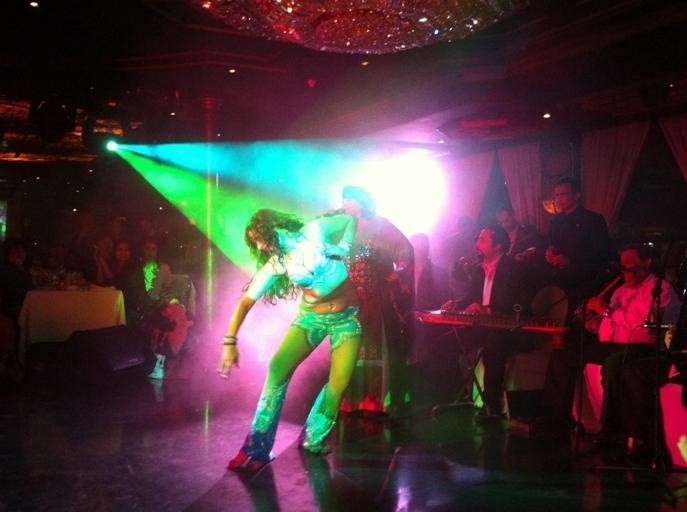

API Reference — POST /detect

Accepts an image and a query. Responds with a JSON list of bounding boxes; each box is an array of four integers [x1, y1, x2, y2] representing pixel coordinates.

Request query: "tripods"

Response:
[[586, 221, 687, 503]]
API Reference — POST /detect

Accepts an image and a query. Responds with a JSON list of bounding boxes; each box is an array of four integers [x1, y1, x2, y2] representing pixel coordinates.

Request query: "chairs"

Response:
[[497, 284, 570, 399]]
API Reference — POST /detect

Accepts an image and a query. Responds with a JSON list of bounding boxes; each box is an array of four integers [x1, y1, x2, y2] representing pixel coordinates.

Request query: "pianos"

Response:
[[413, 307, 569, 334]]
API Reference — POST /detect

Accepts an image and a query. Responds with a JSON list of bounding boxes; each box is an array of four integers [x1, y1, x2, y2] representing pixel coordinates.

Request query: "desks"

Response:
[[17, 278, 126, 376]]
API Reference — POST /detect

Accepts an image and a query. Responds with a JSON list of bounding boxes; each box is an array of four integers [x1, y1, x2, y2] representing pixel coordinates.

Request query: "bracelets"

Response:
[[338, 240, 352, 249], [223, 336, 237, 345]]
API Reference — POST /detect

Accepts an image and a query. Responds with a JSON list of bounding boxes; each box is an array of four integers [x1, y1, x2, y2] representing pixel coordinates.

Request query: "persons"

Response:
[[9, 201, 210, 379], [408, 178, 687, 476], [327, 185, 415, 422], [217, 208, 365, 470]]
